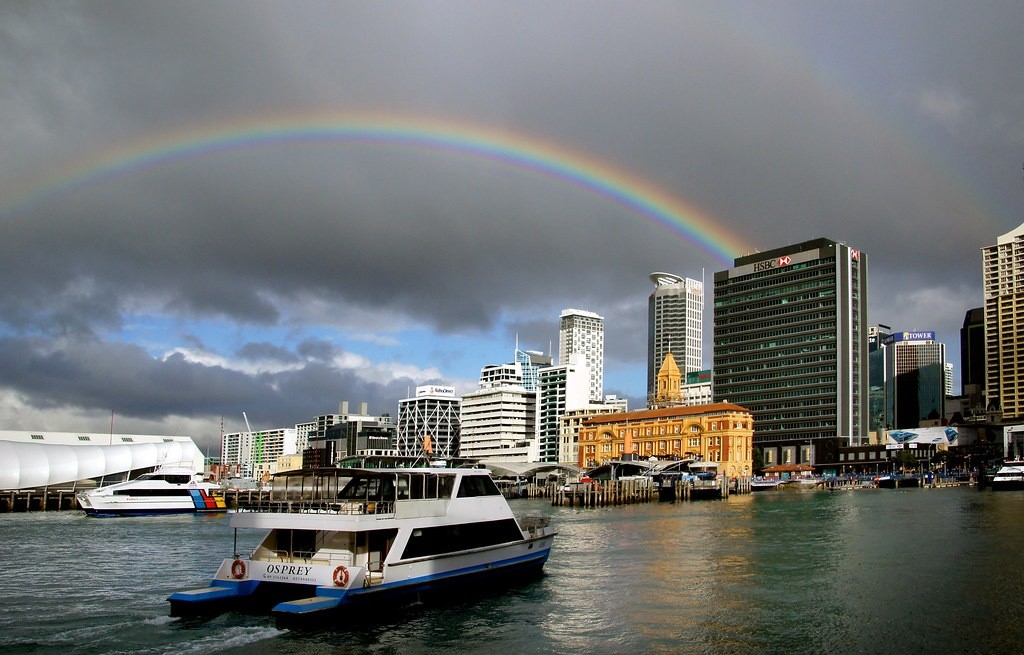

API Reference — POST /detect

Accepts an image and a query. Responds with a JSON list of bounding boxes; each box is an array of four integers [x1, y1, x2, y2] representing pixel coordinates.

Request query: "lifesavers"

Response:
[[231, 559, 246, 579], [333, 566, 349, 586]]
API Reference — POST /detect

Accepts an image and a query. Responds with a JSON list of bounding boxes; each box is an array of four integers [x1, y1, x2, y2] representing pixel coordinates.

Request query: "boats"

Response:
[[673, 461, 731, 499], [76, 465, 230, 517], [163, 466, 560, 634], [750, 476, 785, 489], [993, 459, 1024, 493]]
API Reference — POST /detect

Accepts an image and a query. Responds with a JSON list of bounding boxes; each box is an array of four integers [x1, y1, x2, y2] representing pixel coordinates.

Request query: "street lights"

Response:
[[804, 440, 813, 467], [880, 341, 889, 429]]
